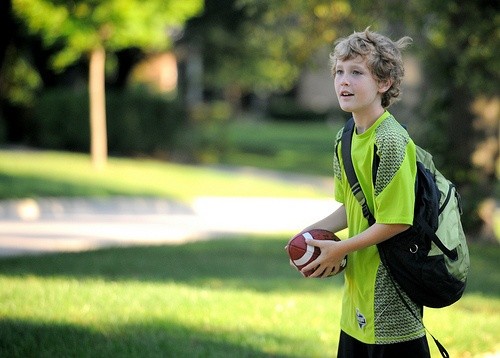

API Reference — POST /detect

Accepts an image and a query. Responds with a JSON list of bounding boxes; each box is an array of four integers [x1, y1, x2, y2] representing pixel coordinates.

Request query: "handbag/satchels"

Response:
[[342, 116, 470, 307]]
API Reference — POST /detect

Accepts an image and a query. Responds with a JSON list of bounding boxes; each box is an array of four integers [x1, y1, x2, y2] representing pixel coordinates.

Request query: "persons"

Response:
[[283, 25, 431, 358]]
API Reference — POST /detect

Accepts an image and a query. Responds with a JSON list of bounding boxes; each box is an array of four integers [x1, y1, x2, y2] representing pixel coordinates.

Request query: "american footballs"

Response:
[[290, 229, 346, 277]]
